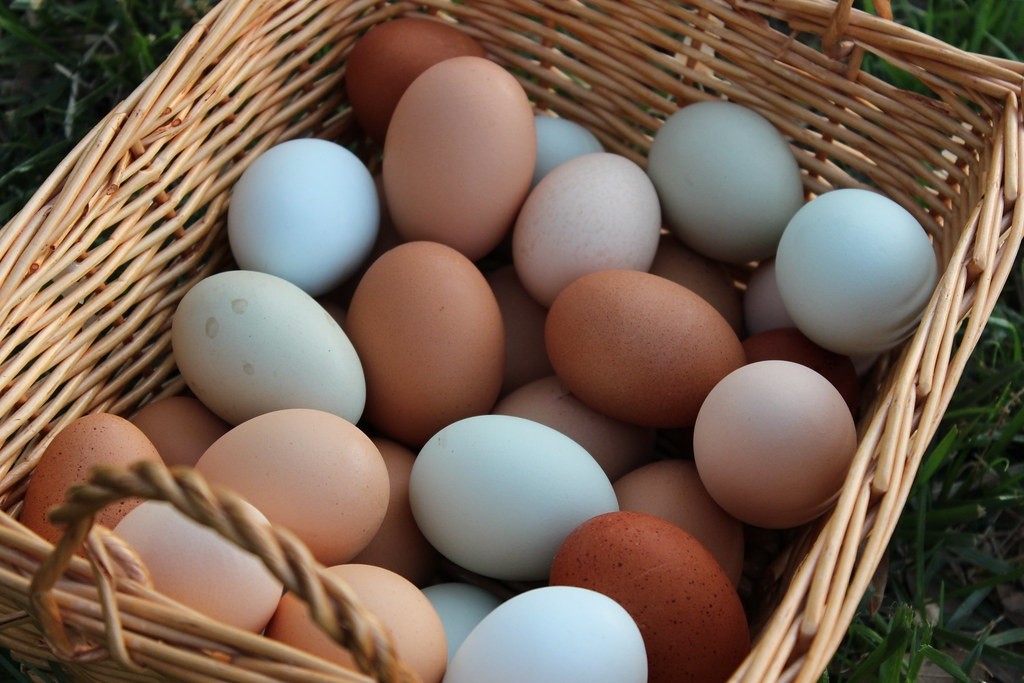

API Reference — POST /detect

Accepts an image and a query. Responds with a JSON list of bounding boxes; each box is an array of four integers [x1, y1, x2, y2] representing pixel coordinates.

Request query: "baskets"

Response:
[[0, 1, 1024, 682]]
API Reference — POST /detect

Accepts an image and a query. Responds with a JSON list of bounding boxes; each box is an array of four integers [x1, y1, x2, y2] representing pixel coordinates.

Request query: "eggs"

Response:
[[26, 15, 934, 683]]
